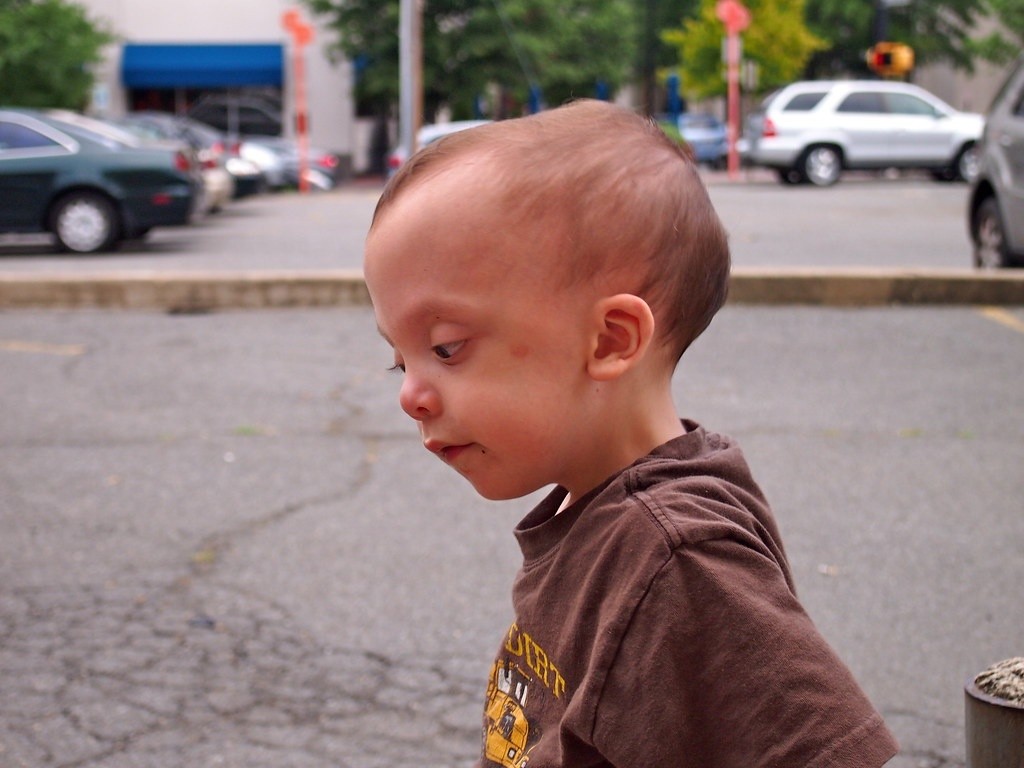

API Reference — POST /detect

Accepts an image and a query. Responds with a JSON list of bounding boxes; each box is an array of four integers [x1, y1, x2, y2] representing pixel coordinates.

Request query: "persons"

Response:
[[364, 97, 900, 768]]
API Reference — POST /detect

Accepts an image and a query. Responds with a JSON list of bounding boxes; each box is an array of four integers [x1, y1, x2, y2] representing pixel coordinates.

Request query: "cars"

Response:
[[674, 114, 736, 168], [166, 120, 235, 187], [41, 103, 234, 224], [390, 118, 497, 188], [251, 136, 348, 193], [965, 59, 1024, 273], [129, 113, 271, 192], [1, 104, 204, 251]]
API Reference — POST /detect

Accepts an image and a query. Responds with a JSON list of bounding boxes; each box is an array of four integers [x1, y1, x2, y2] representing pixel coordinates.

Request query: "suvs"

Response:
[[747, 78, 986, 189]]
